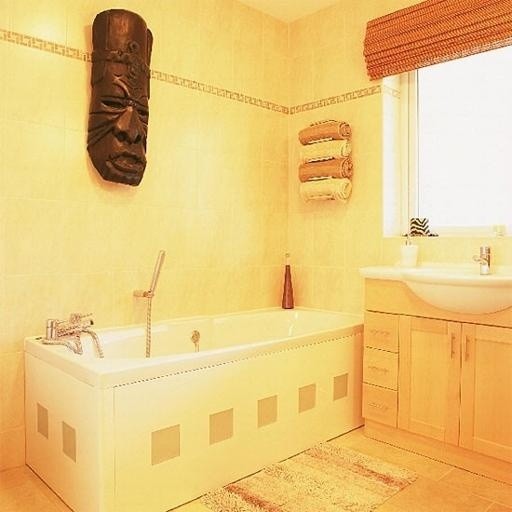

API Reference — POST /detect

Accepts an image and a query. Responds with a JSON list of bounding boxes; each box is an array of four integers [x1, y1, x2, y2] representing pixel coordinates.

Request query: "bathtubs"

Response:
[[23, 304, 365, 376]]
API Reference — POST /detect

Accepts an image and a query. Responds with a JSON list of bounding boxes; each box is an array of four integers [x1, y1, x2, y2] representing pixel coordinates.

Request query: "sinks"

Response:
[[388, 266, 512, 313]]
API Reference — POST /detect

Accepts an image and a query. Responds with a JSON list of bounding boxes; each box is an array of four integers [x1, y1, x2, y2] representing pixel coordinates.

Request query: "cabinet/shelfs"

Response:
[[361, 278, 512, 485]]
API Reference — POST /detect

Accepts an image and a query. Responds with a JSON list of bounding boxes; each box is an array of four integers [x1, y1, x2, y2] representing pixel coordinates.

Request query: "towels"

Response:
[[298, 121, 354, 204]]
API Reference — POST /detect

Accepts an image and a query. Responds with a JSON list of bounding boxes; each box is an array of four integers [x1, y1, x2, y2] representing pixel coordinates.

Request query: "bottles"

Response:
[[282, 264, 294, 309]]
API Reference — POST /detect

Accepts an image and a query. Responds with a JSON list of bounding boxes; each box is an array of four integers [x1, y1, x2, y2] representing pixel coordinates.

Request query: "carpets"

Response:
[[200, 440, 420, 512]]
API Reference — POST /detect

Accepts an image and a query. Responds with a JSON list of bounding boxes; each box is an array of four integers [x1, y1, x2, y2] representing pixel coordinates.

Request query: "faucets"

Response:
[[474, 245, 491, 274], [45, 317, 93, 344]]
